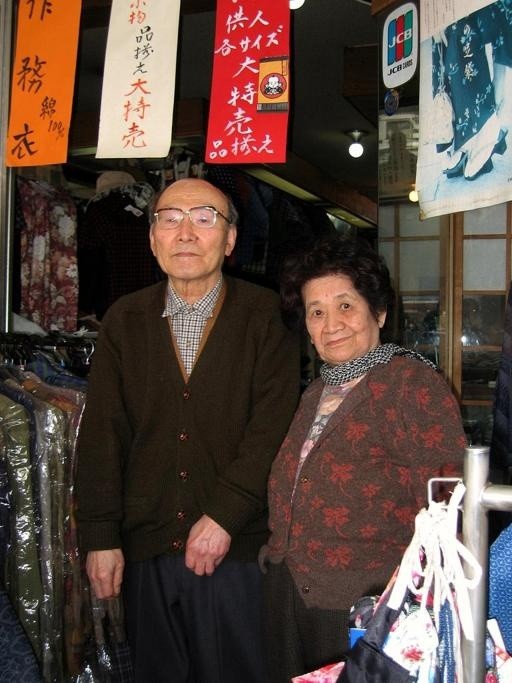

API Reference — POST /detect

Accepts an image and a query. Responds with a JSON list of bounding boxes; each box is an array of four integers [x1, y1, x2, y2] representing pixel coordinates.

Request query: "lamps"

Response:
[[344, 129, 368, 159]]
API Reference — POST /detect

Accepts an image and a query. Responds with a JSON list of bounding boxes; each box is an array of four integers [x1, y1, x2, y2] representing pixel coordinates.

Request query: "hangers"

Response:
[[0, 328, 97, 367], [25, 138, 194, 195]]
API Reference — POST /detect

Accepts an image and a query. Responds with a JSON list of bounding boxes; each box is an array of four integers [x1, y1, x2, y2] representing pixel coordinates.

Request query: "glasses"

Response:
[[150, 205, 231, 229]]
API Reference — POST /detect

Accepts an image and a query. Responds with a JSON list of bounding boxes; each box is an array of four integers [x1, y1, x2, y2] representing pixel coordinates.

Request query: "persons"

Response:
[[259, 221, 469, 682], [73, 174, 303, 683]]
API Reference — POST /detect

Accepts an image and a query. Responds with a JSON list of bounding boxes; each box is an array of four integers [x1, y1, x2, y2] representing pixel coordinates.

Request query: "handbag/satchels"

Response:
[[331, 505, 460, 683]]
